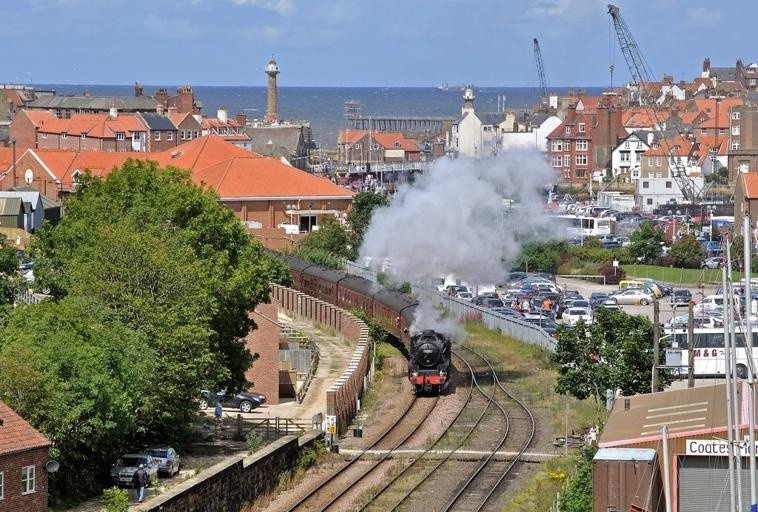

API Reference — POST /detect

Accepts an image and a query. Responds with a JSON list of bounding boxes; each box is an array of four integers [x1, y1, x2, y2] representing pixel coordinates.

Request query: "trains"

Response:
[[281, 257, 452, 396]]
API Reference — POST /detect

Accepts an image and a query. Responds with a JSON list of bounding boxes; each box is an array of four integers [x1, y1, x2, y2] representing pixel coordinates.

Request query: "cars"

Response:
[[111, 453, 158, 489], [571, 231, 628, 249], [334, 171, 387, 196], [557, 201, 690, 226], [198, 387, 267, 412], [667, 277, 758, 327], [444, 271, 691, 325], [698, 218, 732, 269], [144, 445, 180, 478]]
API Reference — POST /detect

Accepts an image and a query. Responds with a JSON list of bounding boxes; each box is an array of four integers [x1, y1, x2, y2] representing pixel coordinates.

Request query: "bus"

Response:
[[550, 215, 614, 240], [659, 326, 758, 379]]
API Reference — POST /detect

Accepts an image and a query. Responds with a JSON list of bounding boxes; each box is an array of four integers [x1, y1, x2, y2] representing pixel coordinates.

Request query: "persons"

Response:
[[131, 462, 152, 502], [213, 397, 226, 432]]
